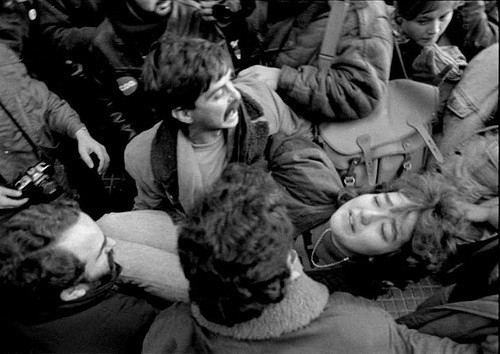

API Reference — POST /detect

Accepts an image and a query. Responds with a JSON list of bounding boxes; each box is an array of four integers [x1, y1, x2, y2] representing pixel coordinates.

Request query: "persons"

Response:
[[0, 0, 500, 354]]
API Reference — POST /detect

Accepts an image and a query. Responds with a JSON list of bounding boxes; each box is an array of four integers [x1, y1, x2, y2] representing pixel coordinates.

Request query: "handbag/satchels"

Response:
[[318, 77, 441, 193]]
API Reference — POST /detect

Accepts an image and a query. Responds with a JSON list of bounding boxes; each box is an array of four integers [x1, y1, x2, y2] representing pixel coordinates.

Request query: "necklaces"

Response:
[[311, 228, 349, 268]]
